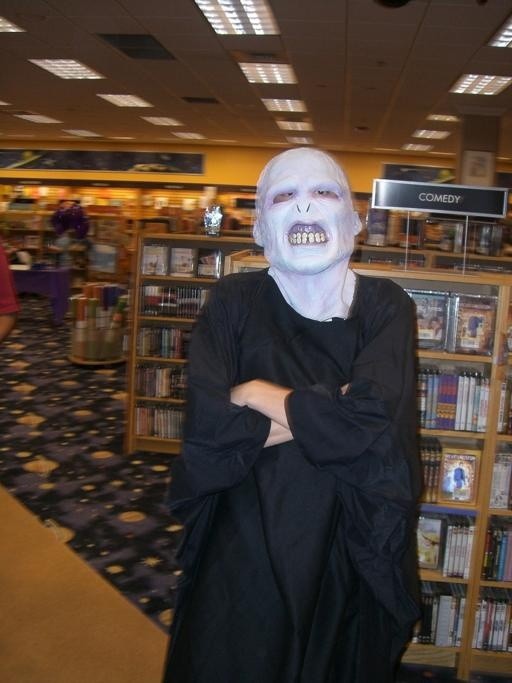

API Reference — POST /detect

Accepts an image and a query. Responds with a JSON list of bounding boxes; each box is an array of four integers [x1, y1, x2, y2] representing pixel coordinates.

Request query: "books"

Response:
[[134, 243, 224, 441], [1, 192, 200, 285], [349, 195, 511, 273], [405, 286, 511, 652]]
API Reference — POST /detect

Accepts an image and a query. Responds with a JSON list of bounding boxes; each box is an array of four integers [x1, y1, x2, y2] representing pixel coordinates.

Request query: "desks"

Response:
[[8, 263, 70, 329]]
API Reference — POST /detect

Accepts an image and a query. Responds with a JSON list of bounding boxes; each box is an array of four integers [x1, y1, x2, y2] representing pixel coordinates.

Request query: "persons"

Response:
[[158, 145, 425, 680], [0, 245, 22, 343]]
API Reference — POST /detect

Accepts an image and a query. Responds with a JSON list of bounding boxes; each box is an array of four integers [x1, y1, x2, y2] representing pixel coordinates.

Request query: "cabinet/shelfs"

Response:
[[221, 246, 510, 682], [0, 178, 372, 282], [125, 227, 510, 459]]
